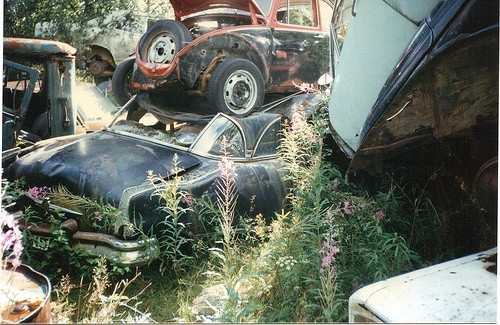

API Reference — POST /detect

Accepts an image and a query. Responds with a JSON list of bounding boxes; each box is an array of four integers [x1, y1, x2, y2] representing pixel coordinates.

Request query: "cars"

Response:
[[34, 11, 158, 78], [1, 93, 330, 268], [317, 0, 497, 215], [346, 248, 497, 324], [111, 0, 336, 117]]
